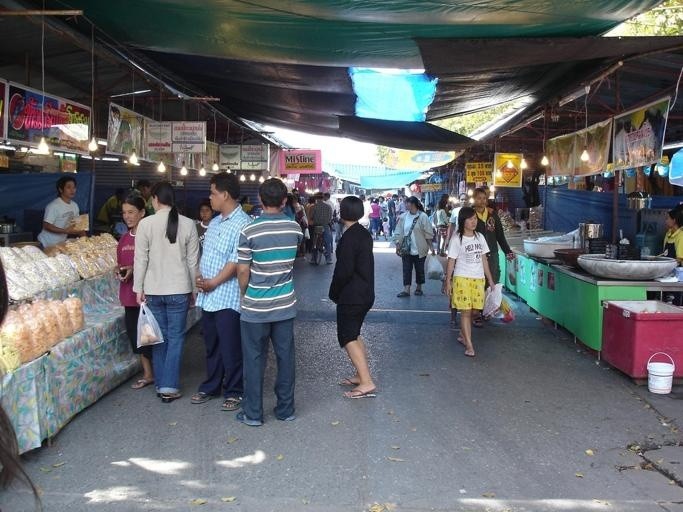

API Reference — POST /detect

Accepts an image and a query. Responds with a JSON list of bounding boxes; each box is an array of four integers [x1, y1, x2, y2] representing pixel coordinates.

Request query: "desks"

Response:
[[498, 245, 682, 362]]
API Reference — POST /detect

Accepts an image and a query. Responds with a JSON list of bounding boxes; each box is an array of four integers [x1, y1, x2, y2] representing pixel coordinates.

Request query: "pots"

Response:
[[625, 191, 653, 211], [0, 222, 14, 234]]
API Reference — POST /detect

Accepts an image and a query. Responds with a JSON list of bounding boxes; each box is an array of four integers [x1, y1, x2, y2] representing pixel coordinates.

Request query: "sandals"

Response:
[[156, 388, 179, 403]]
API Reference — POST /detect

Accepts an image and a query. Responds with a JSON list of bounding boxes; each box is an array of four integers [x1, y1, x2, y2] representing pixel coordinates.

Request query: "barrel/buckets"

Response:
[[646, 351, 675, 397], [578, 221, 605, 255]]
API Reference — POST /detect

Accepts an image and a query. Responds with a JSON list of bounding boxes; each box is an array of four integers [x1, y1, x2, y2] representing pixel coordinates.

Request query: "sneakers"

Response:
[[414, 290, 423, 296], [464, 345, 474, 357], [284, 415, 297, 421], [236, 409, 263, 427], [457, 334, 465, 345], [397, 291, 410, 297]]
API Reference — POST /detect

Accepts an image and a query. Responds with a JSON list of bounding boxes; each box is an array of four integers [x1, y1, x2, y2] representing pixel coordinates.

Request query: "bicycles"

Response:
[[312, 218, 338, 267]]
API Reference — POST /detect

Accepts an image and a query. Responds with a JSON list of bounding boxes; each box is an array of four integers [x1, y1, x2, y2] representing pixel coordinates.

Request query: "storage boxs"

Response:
[[601, 296, 683, 386]]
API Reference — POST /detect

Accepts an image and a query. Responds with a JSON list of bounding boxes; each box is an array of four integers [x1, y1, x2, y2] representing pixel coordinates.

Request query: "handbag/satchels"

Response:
[[482, 282, 505, 319], [396, 236, 411, 257]]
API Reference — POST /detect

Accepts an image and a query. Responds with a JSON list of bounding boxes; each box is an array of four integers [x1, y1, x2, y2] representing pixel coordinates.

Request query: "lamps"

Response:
[[447, 86, 590, 204]]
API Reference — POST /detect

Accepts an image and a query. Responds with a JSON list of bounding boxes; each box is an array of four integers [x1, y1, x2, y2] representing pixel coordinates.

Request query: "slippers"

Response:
[[338, 377, 360, 386], [131, 377, 153, 389], [190, 390, 216, 404], [220, 397, 241, 411], [343, 390, 376, 400]]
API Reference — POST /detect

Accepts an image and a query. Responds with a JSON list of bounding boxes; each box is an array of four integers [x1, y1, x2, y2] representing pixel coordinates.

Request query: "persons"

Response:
[[35, 175, 87, 253], [133, 181, 199, 404], [191, 169, 254, 411], [95, 187, 124, 236], [661, 208, 683, 306], [441, 206, 495, 357], [190, 190, 344, 335], [359, 185, 515, 332], [328, 196, 376, 399], [114, 195, 155, 390], [237, 178, 296, 426], [136, 178, 156, 217]]
